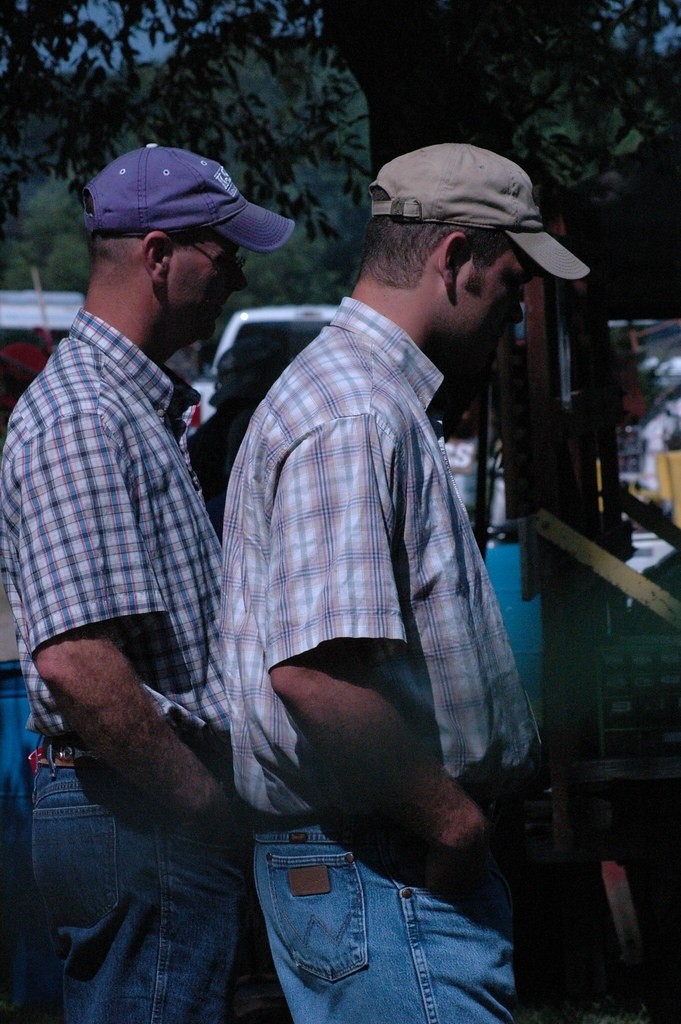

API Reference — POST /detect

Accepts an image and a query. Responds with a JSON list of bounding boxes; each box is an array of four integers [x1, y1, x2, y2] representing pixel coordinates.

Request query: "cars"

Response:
[[0, 278, 681, 577]]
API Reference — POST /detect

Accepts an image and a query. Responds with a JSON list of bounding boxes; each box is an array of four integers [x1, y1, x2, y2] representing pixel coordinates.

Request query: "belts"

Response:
[[35, 736, 75, 768]]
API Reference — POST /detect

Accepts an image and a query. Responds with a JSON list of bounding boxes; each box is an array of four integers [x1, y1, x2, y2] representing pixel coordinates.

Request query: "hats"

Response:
[[368, 143, 592, 279], [81, 141, 295, 254]]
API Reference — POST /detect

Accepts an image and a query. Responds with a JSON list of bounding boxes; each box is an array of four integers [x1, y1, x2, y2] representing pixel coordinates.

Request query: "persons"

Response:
[[1, 141, 296, 1024], [215, 140, 597, 1020]]
